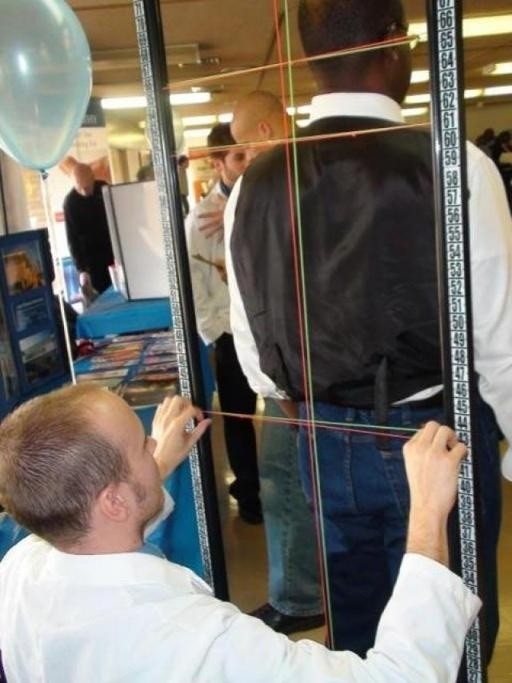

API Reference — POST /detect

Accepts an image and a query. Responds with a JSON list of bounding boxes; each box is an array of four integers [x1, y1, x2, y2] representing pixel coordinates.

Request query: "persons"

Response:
[[474, 129, 512, 220], [3, 252, 45, 294], [56, 160, 115, 298], [0, 378, 489, 681], [135, 87, 327, 637], [220, 1, 512, 681]]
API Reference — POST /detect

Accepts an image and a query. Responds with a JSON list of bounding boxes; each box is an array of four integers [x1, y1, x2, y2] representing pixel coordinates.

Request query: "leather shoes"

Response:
[[245, 603, 327, 634]]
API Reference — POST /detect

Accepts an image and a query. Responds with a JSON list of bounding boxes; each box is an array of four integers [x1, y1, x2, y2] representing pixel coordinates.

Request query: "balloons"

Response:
[[0, 0, 96, 180]]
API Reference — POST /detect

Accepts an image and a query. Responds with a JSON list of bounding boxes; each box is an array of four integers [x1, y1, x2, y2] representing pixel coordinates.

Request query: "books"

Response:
[[68, 325, 180, 412], [1, 233, 51, 298], [9, 295, 56, 332], [15, 325, 68, 383]]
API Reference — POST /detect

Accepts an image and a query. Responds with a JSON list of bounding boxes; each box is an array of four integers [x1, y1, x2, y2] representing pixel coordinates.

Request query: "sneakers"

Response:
[[238, 499, 264, 525]]
